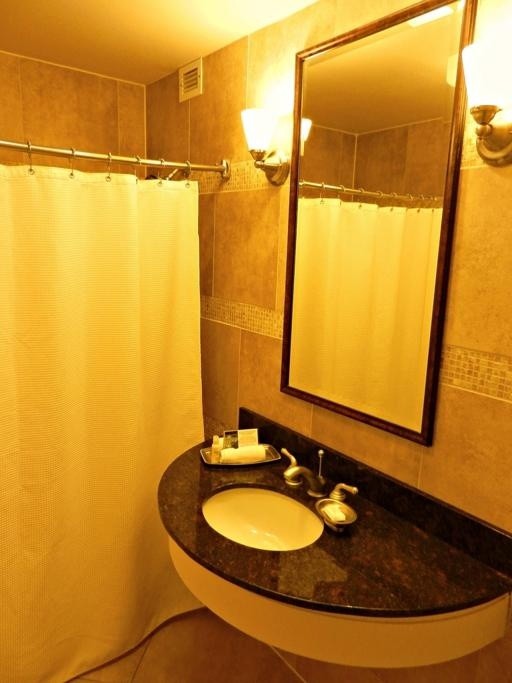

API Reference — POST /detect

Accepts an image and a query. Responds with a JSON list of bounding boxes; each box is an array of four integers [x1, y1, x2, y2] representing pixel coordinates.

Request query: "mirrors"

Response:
[[278, 0, 477, 447]]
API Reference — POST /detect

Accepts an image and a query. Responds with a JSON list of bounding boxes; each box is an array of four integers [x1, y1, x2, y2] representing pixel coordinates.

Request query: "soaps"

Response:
[[323, 502, 346, 523]]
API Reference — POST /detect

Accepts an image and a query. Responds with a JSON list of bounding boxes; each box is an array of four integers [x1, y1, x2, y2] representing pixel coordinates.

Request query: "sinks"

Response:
[[202, 482, 323, 553]]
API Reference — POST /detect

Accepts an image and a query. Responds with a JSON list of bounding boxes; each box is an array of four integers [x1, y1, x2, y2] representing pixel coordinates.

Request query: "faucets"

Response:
[[282, 465, 329, 499]]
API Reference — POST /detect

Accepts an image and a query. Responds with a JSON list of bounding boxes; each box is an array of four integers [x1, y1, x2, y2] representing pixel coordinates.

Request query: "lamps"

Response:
[[460, 33, 512, 167], [239, 105, 291, 185]]
